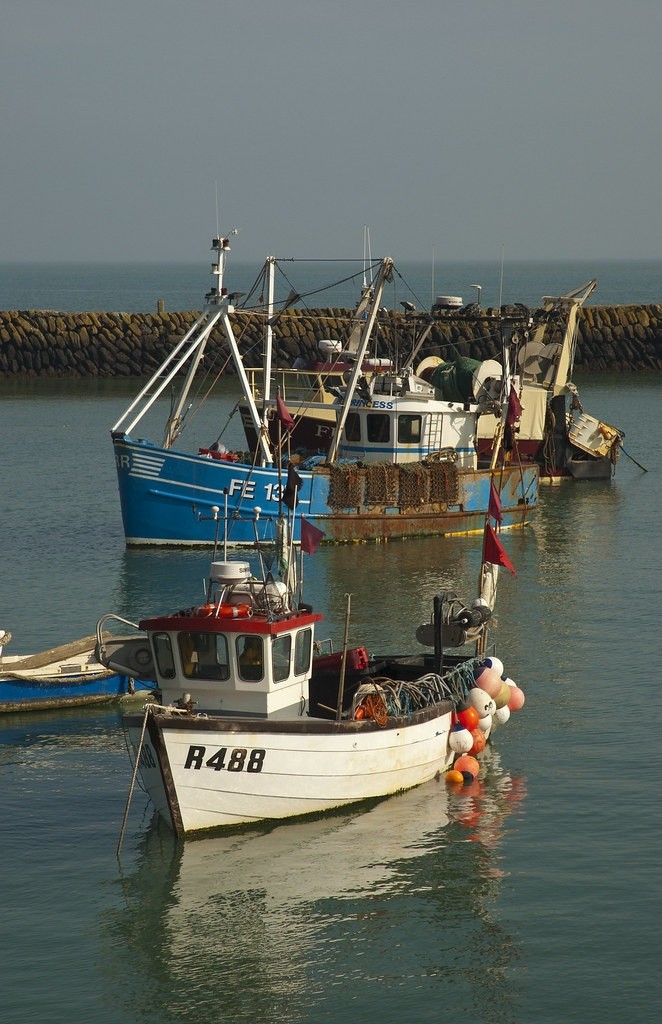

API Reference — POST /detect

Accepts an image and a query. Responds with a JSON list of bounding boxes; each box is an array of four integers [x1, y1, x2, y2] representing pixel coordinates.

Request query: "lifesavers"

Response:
[[349, 704, 375, 720], [193, 602, 249, 618]]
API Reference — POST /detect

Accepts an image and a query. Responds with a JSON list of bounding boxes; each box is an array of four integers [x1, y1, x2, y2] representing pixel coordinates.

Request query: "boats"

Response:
[[0, 630, 159, 715], [110, 179, 627, 550], [92, 382, 512, 839]]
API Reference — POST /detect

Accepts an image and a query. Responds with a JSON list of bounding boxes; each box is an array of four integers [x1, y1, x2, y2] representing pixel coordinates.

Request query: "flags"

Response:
[[485, 523, 517, 577], [276, 395, 296, 426], [488, 480, 503, 535], [301, 518, 325, 556]]
